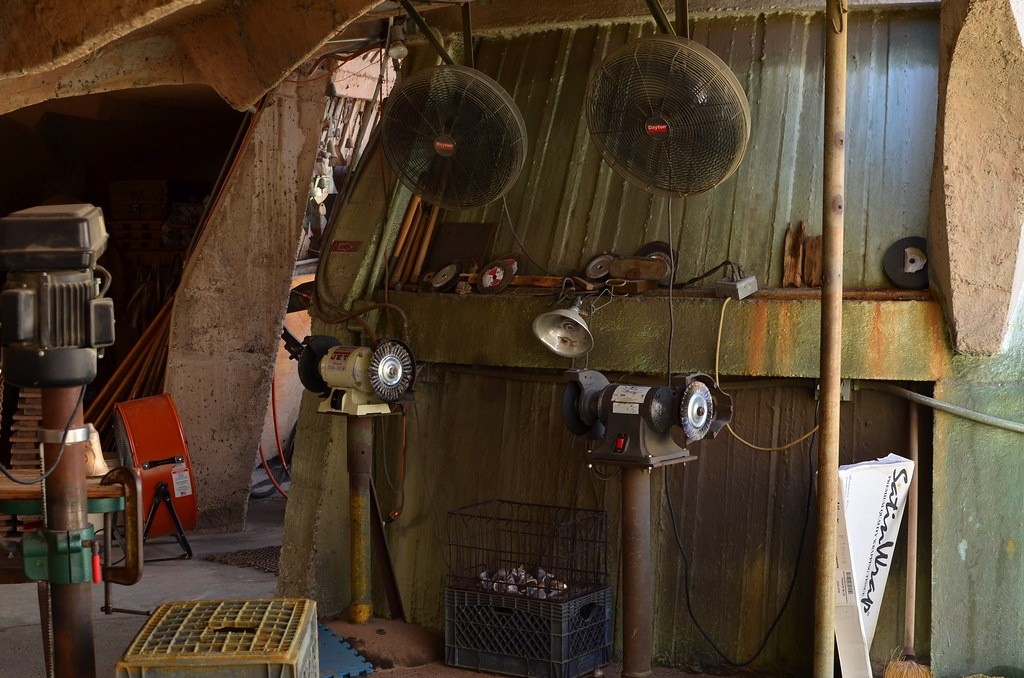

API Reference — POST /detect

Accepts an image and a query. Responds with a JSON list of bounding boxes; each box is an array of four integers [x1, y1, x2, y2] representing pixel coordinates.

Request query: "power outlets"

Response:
[[712, 272, 759, 298]]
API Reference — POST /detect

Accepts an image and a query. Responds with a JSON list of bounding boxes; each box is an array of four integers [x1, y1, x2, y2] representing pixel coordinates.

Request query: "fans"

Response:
[[377, 63, 528, 212], [584, 35, 754, 200]]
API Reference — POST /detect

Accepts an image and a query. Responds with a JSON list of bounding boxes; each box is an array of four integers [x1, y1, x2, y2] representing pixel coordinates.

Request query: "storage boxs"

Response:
[[450, 570, 618, 677], [112, 594, 323, 678]]
